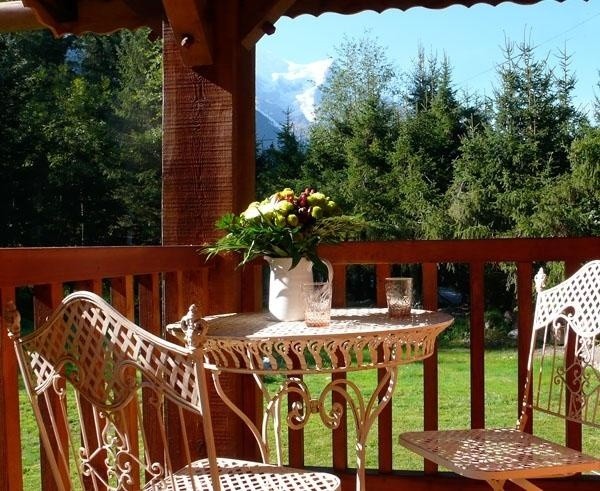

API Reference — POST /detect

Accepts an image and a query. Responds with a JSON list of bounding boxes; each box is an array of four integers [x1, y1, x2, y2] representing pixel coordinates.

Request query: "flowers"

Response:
[[199, 183, 363, 273]]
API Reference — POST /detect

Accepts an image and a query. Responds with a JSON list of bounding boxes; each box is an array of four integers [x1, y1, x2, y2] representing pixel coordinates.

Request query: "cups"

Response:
[[385, 276, 414, 320], [303, 281, 333, 329]]
